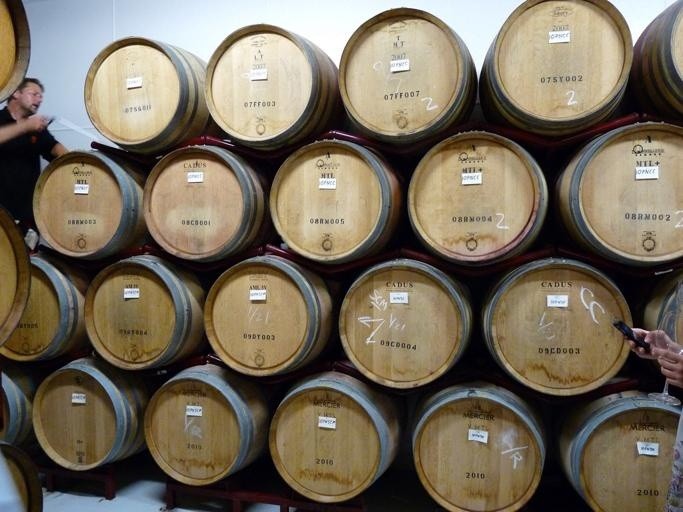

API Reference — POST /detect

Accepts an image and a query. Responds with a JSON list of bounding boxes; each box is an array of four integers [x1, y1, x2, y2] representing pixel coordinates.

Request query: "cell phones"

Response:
[[613, 321, 650, 352]]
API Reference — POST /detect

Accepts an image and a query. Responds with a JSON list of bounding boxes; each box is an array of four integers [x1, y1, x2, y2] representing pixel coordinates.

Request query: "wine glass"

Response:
[[648, 310, 683, 406]]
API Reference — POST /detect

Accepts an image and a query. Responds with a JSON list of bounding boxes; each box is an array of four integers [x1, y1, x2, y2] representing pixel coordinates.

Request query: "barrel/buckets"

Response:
[[1, 0, 683, 511]]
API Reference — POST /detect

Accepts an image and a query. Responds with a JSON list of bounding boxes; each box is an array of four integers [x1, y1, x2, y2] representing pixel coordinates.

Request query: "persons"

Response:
[[0, 79, 69, 235], [625, 326, 682, 511]]
